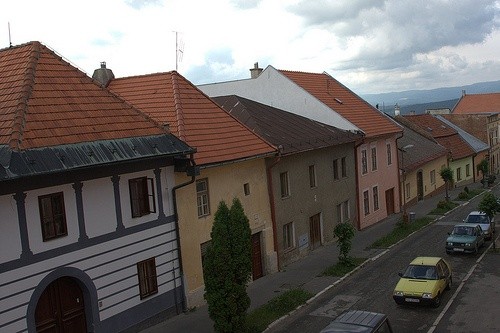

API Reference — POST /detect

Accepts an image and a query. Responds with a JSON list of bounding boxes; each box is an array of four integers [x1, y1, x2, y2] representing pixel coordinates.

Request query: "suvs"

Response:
[[318, 309, 394, 333]]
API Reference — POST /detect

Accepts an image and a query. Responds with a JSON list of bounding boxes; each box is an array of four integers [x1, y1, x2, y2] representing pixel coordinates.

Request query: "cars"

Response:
[[462, 210, 496, 241], [392, 256, 453, 309], [445, 224, 485, 257]]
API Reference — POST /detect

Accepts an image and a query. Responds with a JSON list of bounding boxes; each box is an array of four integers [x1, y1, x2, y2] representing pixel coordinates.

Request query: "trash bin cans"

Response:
[[410, 212, 417, 225]]
